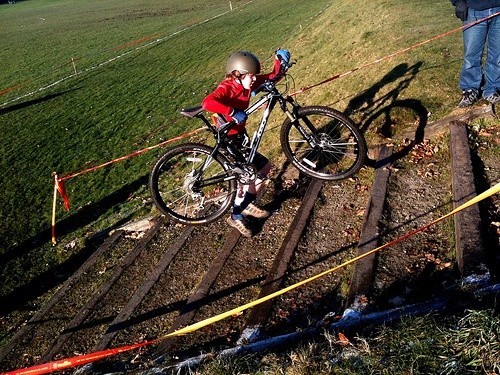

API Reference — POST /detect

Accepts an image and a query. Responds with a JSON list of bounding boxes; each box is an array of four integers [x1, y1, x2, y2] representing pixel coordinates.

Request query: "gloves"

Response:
[[232, 110, 248, 124], [275, 48, 290, 64], [455, 0, 469, 21]]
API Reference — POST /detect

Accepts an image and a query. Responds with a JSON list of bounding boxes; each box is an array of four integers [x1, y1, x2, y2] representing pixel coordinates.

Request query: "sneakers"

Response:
[[226, 217, 253, 237], [458, 89, 479, 108], [483, 92, 500, 104], [240, 202, 270, 219]]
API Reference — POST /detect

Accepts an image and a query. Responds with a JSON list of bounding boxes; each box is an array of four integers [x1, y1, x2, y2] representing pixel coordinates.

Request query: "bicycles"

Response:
[[149, 54, 366, 227]]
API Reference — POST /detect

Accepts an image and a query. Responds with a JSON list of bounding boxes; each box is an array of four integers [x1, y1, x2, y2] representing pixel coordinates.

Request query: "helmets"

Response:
[[225, 51, 260, 76]]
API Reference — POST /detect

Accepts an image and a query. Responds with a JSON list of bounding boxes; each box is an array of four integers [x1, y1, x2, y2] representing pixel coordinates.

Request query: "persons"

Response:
[[450, 0, 500, 108], [202, 49, 291, 238]]
